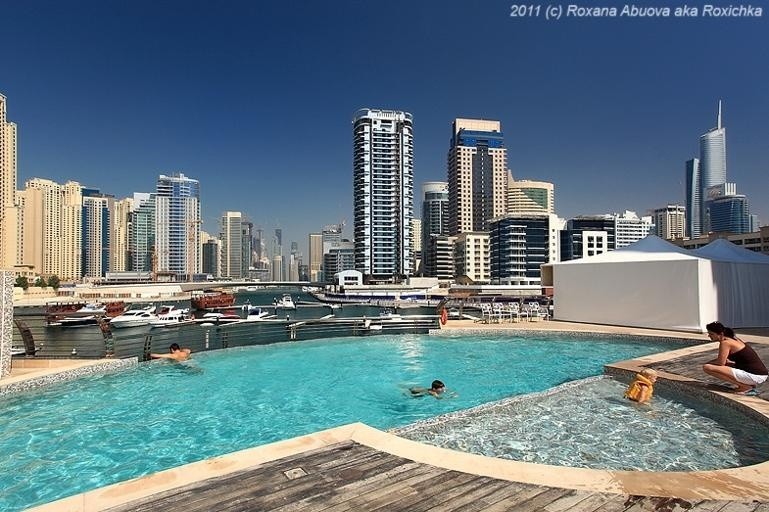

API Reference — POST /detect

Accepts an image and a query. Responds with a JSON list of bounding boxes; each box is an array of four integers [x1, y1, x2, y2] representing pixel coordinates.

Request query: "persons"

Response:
[[406, 380, 456, 399], [703, 321, 769, 396], [623, 368, 658, 417], [149, 344, 203, 375]]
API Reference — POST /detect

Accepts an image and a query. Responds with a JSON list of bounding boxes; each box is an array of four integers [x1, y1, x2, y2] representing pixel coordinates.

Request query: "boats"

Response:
[[45, 288, 300, 330], [304, 280, 553, 309]]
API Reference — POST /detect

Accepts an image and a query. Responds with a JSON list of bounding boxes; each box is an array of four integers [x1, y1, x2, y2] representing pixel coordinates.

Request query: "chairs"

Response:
[[474, 301, 550, 324]]
